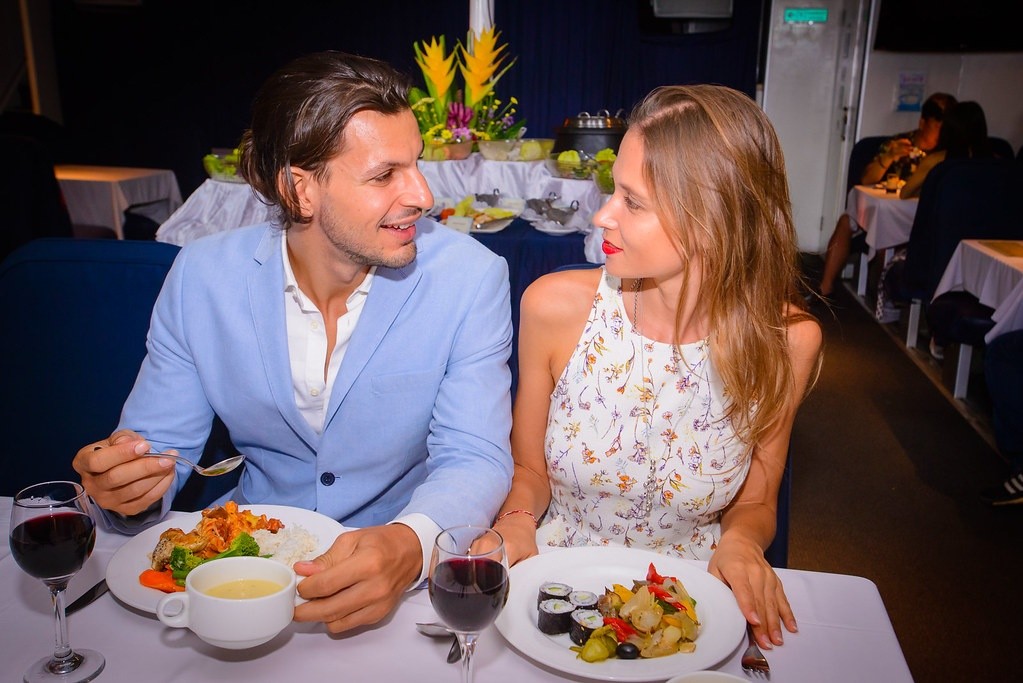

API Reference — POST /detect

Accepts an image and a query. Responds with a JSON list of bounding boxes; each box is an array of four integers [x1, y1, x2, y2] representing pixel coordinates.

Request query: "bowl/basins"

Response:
[[204, 158, 248, 183], [475, 138, 556, 162], [544, 152, 596, 180], [422, 141, 473, 160], [591, 173, 615, 195]]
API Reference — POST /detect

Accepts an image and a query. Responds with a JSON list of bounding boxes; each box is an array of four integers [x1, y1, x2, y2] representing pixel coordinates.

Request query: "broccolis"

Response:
[[170, 531, 271, 587]]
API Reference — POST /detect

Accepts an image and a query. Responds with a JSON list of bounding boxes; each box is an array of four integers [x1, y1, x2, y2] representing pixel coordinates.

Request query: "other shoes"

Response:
[[978, 472, 1023, 508], [803, 287, 834, 306], [929, 336, 945, 359]]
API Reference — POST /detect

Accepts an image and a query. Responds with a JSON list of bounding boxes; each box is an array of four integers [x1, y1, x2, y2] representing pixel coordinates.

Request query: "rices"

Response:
[[252, 522, 317, 572]]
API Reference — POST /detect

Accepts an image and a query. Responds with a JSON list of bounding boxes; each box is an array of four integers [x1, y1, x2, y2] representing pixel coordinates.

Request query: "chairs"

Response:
[[850, 137, 883, 182], [0, 239, 245, 511]]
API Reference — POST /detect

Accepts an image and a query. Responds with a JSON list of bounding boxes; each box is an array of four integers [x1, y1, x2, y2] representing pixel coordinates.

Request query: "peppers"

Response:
[[601, 562, 697, 642]]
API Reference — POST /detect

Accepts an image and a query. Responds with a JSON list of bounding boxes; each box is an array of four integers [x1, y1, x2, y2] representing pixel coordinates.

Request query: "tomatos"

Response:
[[440, 208, 455, 220]]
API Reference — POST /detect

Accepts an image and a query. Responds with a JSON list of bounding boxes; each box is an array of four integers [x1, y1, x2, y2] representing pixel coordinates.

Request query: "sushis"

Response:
[[536, 582, 604, 645]]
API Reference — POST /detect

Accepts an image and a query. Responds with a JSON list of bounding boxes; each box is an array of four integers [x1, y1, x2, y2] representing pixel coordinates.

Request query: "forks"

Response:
[[740, 621, 771, 674]]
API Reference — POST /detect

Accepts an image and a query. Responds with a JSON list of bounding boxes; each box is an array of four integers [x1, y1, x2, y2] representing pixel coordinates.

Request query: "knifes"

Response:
[[65, 577, 109, 616], [446, 638, 461, 664]]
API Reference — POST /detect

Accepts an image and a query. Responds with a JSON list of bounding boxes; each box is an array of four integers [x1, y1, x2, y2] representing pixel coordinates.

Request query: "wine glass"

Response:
[[428, 525, 510, 682], [7, 480, 106, 683]]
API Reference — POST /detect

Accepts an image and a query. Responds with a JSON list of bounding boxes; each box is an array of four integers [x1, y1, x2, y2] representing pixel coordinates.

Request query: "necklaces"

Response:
[[630, 276, 707, 513]]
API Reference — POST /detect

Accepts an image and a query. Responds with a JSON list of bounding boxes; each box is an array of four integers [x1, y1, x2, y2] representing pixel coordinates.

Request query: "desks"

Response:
[[2, 497, 917, 683], [847, 182, 921, 319], [52, 164, 185, 242], [929, 238, 1023, 403], [157, 157, 604, 394]]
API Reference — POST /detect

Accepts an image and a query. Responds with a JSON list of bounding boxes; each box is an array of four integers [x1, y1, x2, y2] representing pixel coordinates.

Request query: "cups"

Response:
[[474, 188, 500, 207], [541, 200, 580, 225], [665, 671, 750, 683], [156, 556, 297, 651], [886, 173, 899, 194], [526, 191, 561, 214]]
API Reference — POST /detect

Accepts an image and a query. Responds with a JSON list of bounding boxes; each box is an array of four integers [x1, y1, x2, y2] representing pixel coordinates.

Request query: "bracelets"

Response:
[[490, 510, 538, 531]]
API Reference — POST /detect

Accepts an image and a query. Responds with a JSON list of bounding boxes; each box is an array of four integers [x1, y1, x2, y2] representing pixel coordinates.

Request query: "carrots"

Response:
[[139, 568, 185, 593]]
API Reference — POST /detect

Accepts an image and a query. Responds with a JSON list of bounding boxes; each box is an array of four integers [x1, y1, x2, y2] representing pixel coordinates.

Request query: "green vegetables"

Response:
[[555, 147, 619, 193], [204, 148, 242, 177], [455, 195, 514, 222]]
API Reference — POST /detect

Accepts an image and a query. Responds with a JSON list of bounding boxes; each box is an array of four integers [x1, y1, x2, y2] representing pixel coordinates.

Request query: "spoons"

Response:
[[414, 621, 455, 637], [94, 447, 246, 477]]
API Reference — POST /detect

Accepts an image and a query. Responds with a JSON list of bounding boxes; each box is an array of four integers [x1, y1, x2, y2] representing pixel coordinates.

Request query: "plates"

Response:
[[106, 504, 349, 619], [438, 199, 579, 236], [494, 546, 747, 682], [881, 181, 904, 189]]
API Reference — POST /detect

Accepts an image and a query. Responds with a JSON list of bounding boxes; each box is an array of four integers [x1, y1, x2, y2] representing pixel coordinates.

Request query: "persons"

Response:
[[70, 48, 516, 634], [464, 85, 824, 650], [804, 91, 1023, 329]]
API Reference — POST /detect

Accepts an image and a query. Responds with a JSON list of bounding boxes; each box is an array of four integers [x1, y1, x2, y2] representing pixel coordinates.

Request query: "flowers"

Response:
[[402, 26, 530, 144]]
